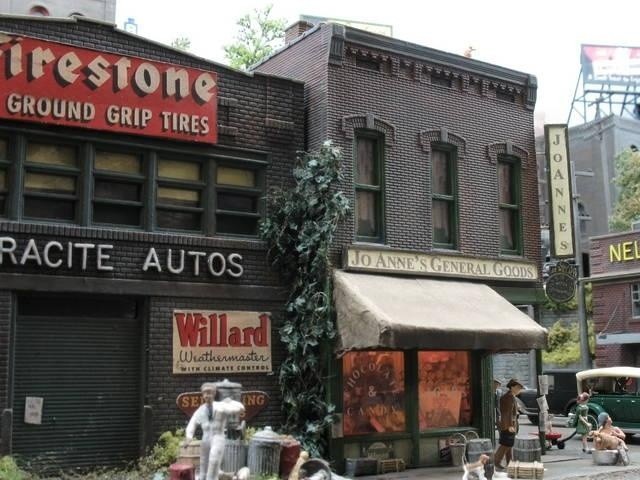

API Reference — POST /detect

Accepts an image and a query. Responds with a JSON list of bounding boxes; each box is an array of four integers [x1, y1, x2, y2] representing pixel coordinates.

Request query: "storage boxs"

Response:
[[507, 459, 544, 479], [344, 458, 378, 477]]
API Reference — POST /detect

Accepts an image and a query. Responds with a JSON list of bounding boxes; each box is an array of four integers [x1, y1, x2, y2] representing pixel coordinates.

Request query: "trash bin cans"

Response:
[[222, 425, 283, 478]]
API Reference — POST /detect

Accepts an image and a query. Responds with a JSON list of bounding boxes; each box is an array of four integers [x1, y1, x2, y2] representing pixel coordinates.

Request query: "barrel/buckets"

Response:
[[512, 435, 541, 463], [468, 439, 495, 480]]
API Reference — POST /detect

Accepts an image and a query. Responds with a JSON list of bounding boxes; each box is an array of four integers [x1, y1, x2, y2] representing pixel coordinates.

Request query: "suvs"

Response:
[[573, 364, 640, 444], [514, 368, 587, 427]]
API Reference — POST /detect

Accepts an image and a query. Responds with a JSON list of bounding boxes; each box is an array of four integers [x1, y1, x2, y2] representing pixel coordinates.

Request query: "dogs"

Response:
[[460, 454, 490, 480]]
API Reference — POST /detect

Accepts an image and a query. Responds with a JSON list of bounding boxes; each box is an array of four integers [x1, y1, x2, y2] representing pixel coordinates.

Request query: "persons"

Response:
[[494, 378, 523, 469], [573, 392, 592, 454], [184, 382, 244, 480], [594, 412, 626, 465]]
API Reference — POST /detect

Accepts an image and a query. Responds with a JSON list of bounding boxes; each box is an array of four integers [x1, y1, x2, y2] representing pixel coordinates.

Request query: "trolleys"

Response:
[[529, 426, 577, 450]]
